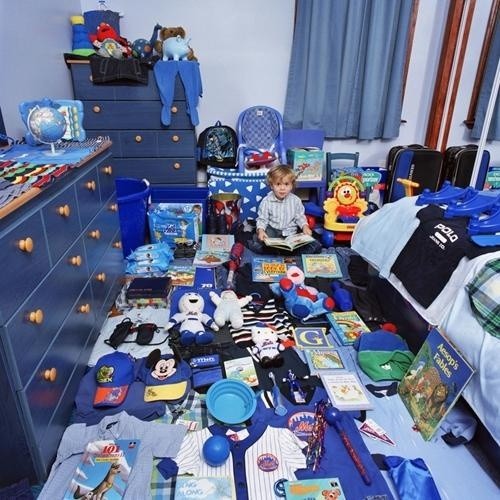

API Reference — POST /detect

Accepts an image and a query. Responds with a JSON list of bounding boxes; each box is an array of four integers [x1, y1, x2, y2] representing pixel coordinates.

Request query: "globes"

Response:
[[27, 105, 66, 156]]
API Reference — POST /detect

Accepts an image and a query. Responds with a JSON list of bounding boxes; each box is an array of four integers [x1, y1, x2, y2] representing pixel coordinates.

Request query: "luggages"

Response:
[[383, 144, 490, 204]]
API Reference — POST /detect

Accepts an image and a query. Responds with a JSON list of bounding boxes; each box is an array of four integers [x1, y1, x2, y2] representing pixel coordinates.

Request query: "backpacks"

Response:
[[195, 120, 238, 168]]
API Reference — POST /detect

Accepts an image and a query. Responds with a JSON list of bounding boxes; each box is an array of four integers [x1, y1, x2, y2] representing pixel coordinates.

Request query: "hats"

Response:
[[143, 354, 192, 403], [92, 352, 134, 409]]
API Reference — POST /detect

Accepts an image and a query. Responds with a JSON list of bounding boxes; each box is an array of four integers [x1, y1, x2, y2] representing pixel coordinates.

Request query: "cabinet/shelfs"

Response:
[[67, 60, 197, 188], [0, 141, 127, 487]]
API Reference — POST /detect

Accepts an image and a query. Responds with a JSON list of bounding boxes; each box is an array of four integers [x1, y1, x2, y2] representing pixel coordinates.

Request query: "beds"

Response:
[[352, 196, 500, 466]]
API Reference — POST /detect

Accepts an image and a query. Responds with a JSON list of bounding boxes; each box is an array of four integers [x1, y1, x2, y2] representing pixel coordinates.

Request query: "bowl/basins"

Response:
[[206, 379, 257, 425]]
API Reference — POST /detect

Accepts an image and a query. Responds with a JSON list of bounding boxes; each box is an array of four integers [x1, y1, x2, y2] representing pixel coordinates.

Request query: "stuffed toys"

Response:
[[208, 289, 254, 330], [245, 322, 286, 369], [267, 265, 335, 322], [162, 292, 221, 346], [86, 20, 198, 62], [282, 367, 311, 403]]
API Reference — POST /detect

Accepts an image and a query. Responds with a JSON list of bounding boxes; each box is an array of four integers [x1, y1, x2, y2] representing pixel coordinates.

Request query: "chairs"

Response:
[[326, 152, 366, 199], [238, 106, 286, 173], [284, 129, 327, 206]]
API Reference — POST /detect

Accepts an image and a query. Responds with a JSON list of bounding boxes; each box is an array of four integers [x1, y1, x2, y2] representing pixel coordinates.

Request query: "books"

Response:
[[160, 265, 197, 287], [192, 249, 236, 270], [63, 439, 140, 500], [291, 325, 334, 349], [282, 477, 347, 499], [325, 310, 374, 346], [173, 475, 235, 500], [251, 255, 289, 284], [264, 232, 317, 253], [201, 232, 236, 253], [223, 356, 260, 388], [395, 325, 476, 442]]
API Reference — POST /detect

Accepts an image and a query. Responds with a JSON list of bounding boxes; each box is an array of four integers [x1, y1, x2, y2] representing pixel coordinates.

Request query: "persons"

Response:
[[246, 163, 323, 256]]
